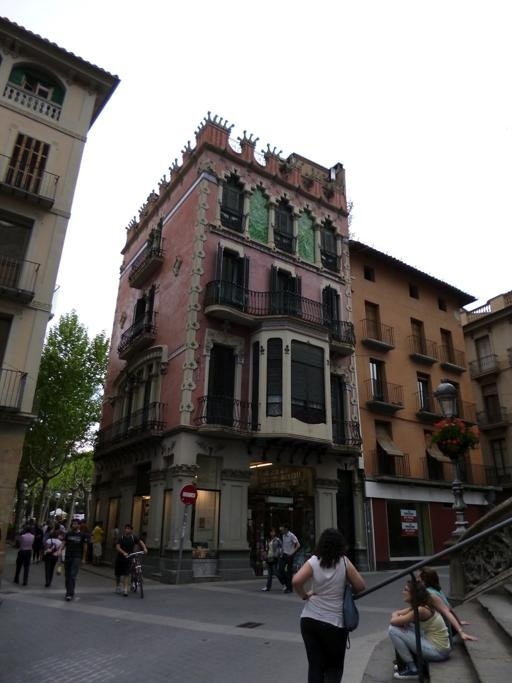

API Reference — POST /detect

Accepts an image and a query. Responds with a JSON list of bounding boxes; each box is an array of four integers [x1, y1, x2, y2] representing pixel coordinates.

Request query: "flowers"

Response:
[[428, 417, 480, 456]]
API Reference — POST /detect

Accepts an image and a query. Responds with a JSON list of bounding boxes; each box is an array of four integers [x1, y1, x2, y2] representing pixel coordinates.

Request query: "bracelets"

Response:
[[457, 628, 463, 632]]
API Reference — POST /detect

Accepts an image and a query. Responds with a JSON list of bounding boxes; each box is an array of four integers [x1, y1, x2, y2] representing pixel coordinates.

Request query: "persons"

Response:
[[275, 524, 300, 592], [12, 511, 105, 600], [114, 525, 148, 597], [387, 583, 451, 680], [417, 568, 479, 641], [261, 528, 288, 592], [291, 529, 367, 682]]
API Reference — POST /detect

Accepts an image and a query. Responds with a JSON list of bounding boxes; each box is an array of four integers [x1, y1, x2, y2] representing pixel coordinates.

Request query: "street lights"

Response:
[[14, 478, 80, 527], [434, 381, 470, 534]]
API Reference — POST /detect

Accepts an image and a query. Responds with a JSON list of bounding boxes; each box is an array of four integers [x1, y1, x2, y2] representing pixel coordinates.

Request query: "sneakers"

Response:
[[393, 668, 420, 680], [393, 663, 408, 672]]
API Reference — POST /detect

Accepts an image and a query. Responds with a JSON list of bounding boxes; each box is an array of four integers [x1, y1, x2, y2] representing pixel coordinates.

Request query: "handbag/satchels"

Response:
[[343, 583, 359, 632]]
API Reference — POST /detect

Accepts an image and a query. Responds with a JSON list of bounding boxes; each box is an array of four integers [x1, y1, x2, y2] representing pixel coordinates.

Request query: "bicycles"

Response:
[[125, 549, 146, 599]]
